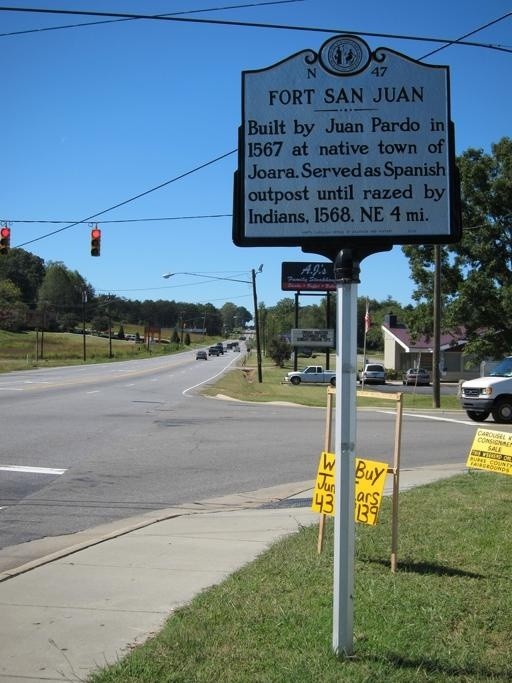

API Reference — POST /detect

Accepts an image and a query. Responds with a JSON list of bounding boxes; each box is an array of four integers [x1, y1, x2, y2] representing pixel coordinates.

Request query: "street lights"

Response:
[[162, 268, 264, 384]]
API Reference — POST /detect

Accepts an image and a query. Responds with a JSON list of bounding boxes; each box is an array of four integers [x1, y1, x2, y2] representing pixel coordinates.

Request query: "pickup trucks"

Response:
[[283, 364, 336, 387]]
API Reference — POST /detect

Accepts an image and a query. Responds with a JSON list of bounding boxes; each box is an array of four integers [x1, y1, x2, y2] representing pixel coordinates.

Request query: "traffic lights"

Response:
[[90, 229, 101, 257], [0, 225, 11, 255]]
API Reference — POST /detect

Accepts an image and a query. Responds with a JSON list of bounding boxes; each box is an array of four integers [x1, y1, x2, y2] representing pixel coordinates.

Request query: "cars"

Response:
[[459, 355, 512, 424], [358, 363, 387, 385], [402, 367, 431, 386], [195, 336, 247, 362]]
